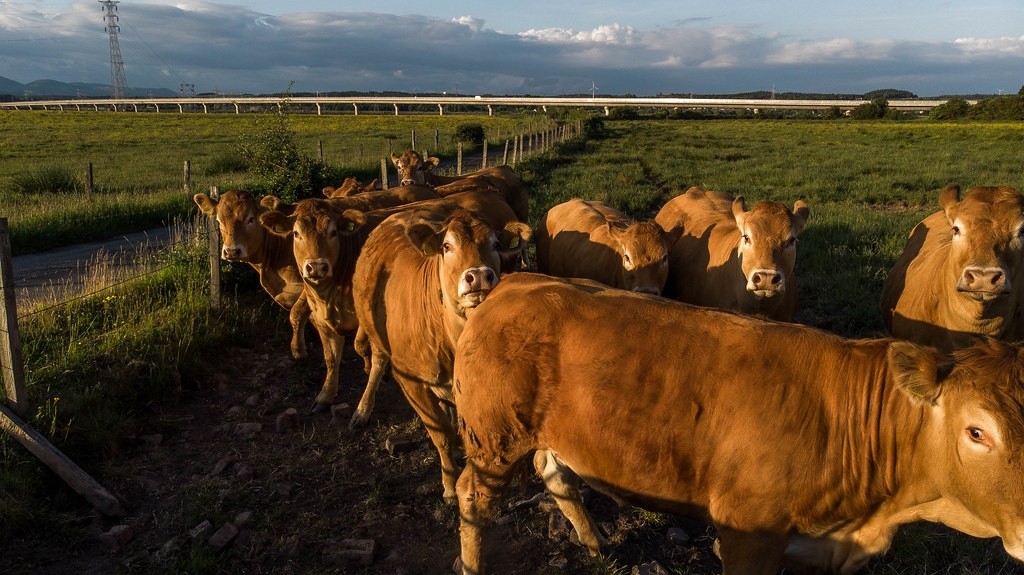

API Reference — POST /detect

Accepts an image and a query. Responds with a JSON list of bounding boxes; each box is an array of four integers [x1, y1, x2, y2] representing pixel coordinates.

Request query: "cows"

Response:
[[193, 149, 1024, 575]]
